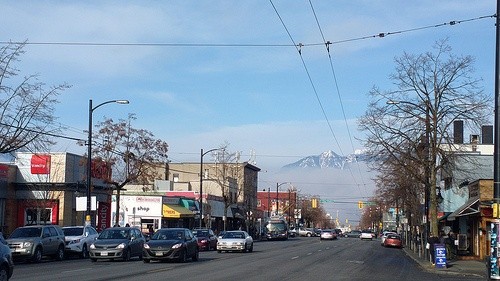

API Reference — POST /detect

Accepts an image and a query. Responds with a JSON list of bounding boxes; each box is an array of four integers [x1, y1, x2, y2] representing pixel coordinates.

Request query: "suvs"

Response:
[[7, 224, 65, 262]]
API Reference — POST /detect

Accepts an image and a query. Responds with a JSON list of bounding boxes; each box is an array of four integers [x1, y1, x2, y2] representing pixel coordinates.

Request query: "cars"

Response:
[[383, 234, 402, 249], [217, 230, 254, 253], [59, 225, 98, 257], [89, 227, 146, 262], [0, 232, 15, 281], [290, 224, 379, 241], [192, 228, 218, 251], [381, 232, 397, 245], [143, 228, 200, 263]]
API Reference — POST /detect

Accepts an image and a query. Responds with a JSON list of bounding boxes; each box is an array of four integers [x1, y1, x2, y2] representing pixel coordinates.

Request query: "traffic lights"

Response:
[[311, 198, 319, 209], [379, 222, 383, 230], [358, 200, 363, 209]]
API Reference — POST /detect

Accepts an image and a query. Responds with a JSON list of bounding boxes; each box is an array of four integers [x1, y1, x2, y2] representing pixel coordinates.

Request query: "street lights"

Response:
[[276, 182, 287, 212], [262, 188, 270, 217], [199, 148, 225, 228], [386, 100, 431, 260], [85, 99, 129, 226]]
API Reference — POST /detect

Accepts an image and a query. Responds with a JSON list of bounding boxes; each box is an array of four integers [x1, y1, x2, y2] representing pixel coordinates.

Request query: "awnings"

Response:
[[195, 202, 211, 219], [162, 203, 194, 218], [181, 199, 200, 211], [438, 199, 481, 222], [208, 200, 233, 218]]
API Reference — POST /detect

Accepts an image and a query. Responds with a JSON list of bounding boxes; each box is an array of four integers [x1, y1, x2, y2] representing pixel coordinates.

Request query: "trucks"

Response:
[[265, 220, 290, 240]]
